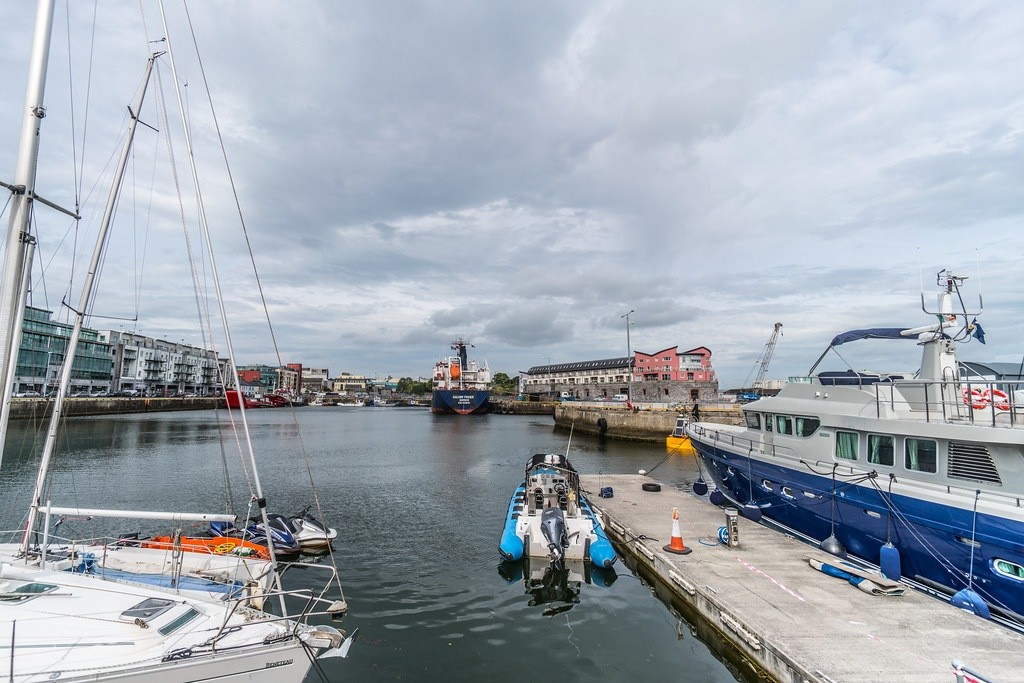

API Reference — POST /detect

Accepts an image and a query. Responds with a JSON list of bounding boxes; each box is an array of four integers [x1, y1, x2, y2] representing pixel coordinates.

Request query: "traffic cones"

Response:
[[661, 507, 694, 556]]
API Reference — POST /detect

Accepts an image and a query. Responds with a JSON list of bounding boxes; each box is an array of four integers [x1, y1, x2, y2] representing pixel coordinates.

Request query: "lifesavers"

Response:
[[962, 390, 988, 409], [984, 389, 1008, 406]]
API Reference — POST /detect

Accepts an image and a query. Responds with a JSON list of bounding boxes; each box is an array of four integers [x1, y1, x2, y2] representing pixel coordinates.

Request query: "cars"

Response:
[[13, 385, 223, 401]]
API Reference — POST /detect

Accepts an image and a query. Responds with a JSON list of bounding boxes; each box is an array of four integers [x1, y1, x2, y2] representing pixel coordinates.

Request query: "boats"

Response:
[[223, 378, 431, 410], [497, 453, 618, 569], [683, 264, 1024, 629], [430, 334, 492, 415], [4, 0, 361, 680]]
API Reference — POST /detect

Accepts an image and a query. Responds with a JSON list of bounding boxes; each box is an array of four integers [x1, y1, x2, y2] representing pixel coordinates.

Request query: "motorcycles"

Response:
[[193, 505, 339, 558]]
